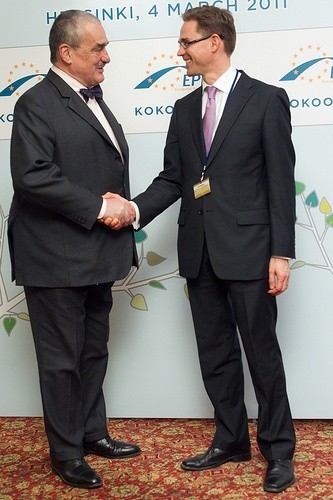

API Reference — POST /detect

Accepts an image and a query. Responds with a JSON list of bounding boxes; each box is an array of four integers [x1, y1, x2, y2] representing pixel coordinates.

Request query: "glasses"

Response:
[[178, 33, 224, 50]]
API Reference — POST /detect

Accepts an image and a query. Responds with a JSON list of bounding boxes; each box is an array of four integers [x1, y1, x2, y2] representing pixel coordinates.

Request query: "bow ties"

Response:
[[80, 83, 103, 103]]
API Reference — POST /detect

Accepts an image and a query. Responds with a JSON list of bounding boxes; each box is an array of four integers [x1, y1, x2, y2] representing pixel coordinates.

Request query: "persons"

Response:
[[100, 6, 296, 492], [7, 11, 141, 488]]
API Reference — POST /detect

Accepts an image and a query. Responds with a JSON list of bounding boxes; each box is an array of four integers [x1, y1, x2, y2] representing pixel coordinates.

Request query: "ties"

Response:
[[203, 86, 218, 157]]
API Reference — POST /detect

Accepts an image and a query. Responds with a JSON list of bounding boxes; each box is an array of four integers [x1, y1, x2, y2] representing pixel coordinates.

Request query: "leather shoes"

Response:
[[83, 436, 142, 459], [181, 446, 251, 471], [263, 458, 295, 493], [51, 457, 103, 489]]
